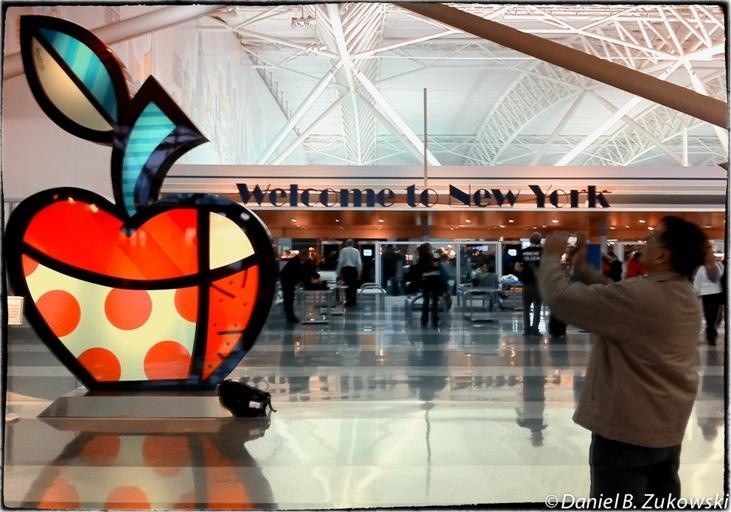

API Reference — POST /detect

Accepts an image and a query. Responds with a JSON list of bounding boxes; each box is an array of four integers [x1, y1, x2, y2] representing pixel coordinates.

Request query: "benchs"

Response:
[[275, 282, 501, 326]]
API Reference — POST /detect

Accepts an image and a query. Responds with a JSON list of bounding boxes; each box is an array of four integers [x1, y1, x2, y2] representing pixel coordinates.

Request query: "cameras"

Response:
[[566, 236, 579, 247]]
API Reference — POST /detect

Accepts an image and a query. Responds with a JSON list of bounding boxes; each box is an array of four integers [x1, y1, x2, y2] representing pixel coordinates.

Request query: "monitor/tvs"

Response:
[[363, 248, 374, 258]]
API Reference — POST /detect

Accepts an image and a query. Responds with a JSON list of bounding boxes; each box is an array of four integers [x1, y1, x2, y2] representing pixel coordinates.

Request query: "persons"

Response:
[[382, 244, 403, 295], [415, 328, 448, 400], [337, 239, 364, 308], [697, 345, 726, 439], [537, 214, 712, 506], [694, 244, 726, 345], [280, 246, 323, 323], [279, 323, 317, 402], [516, 336, 551, 447], [514, 233, 545, 337], [423, 242, 439, 259], [415, 245, 447, 328], [602, 250, 647, 282], [338, 306, 363, 375]]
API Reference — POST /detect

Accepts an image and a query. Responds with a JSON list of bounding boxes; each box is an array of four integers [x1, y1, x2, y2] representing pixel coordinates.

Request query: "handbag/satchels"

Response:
[[216, 419, 271, 449], [219, 379, 276, 417]]
